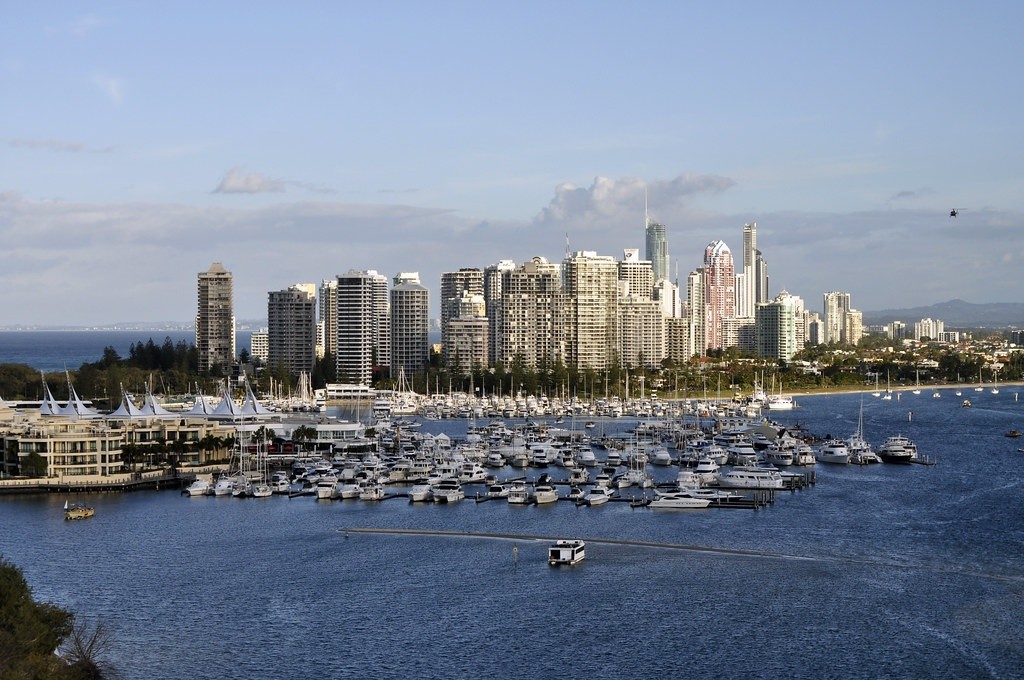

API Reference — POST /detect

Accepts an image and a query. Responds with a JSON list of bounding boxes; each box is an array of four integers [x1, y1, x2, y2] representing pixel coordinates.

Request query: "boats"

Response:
[[961, 400, 971, 409], [487, 484, 509, 497], [531, 472, 559, 503], [433, 477, 465, 504], [652, 473, 744, 503], [315, 473, 337, 499], [692, 459, 721, 486], [646, 496, 713, 508], [359, 479, 385, 500], [723, 470, 786, 491], [407, 478, 438, 502], [185, 478, 213, 496], [880, 436, 918, 459], [569, 486, 586, 499], [548, 538, 586, 567], [583, 486, 611, 506], [878, 442, 913, 465], [215, 475, 235, 496], [337, 479, 363, 498], [64, 499, 95, 520], [507, 480, 529, 503], [272, 480, 289, 493], [1005, 430, 1022, 438]]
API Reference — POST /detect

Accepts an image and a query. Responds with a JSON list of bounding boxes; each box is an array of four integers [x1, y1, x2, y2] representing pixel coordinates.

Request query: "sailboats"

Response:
[[847, 390, 878, 464], [232, 410, 252, 497], [272, 365, 849, 480], [253, 424, 272, 497], [872, 366, 1000, 399]]
